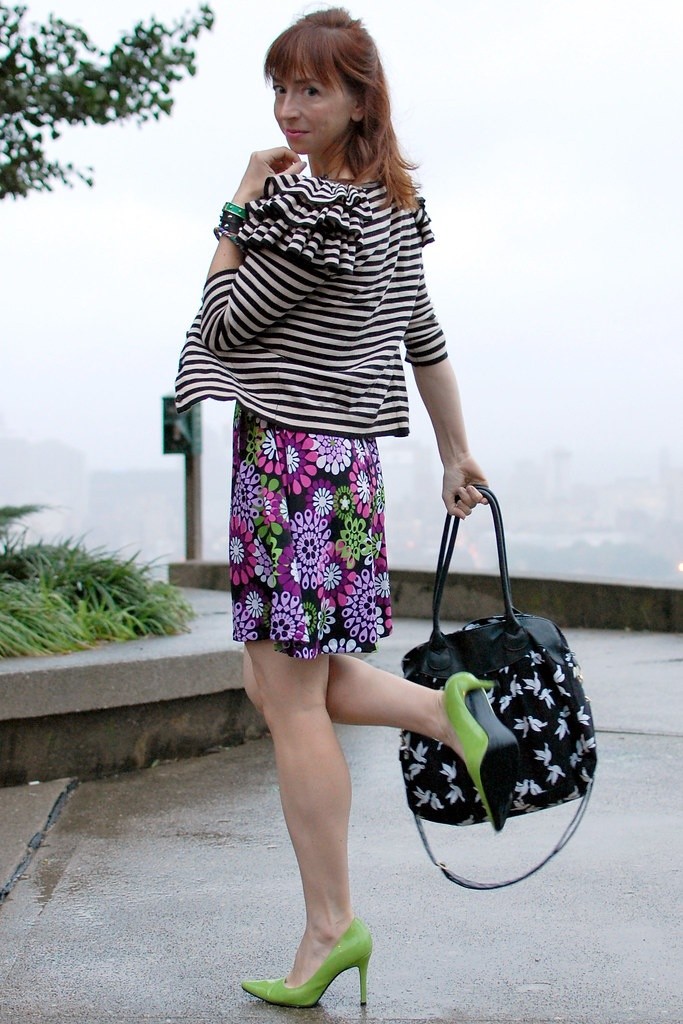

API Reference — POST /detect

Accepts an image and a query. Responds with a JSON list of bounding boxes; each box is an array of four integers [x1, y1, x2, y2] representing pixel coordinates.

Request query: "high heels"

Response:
[[442, 670, 523, 832], [240, 917, 372, 1010]]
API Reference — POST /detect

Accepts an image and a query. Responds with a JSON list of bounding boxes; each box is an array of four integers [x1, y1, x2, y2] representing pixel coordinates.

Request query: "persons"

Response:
[[195, 5, 519, 1008]]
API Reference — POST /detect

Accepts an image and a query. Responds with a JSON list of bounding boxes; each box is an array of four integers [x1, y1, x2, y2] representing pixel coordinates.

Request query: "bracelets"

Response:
[[222, 201, 248, 222], [218, 212, 244, 234], [212, 228, 247, 256]]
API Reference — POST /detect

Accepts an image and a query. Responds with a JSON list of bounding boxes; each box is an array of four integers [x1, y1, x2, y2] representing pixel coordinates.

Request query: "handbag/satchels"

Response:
[[398, 484, 599, 891]]
